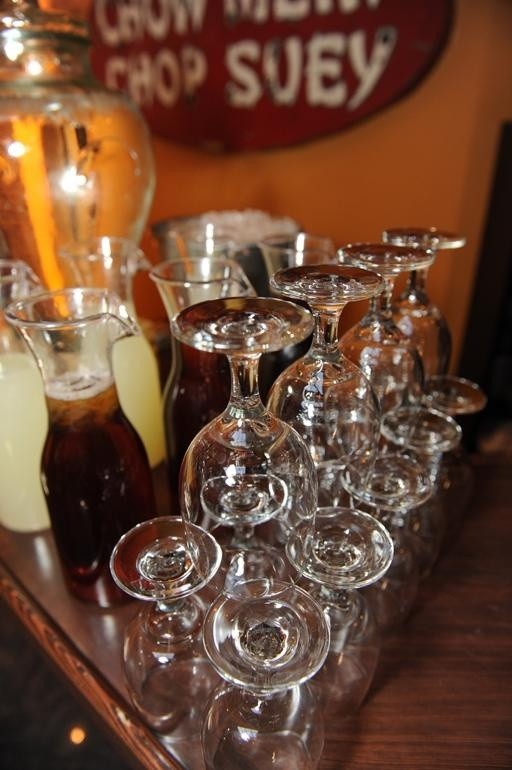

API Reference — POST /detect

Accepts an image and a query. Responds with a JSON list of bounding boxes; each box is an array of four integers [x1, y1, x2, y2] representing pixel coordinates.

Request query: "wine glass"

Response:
[[274, 261, 381, 513], [172, 306, 320, 587], [343, 238, 429, 418], [108, 505, 390, 762], [346, 371, 490, 630]]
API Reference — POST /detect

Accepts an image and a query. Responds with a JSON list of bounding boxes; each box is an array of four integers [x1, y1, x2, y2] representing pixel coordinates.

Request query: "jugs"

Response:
[[2, 236, 353, 609], [2, 0, 175, 541]]
